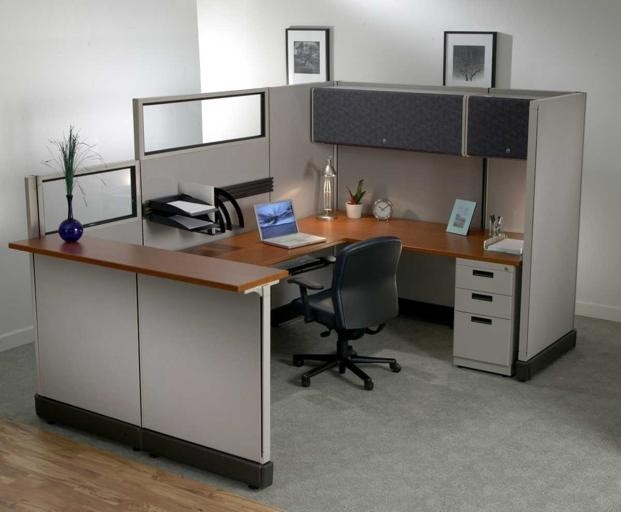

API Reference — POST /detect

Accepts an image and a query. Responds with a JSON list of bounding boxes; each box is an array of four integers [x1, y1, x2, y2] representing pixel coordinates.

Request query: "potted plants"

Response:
[[41, 125, 107, 243], [345, 178, 367, 219]]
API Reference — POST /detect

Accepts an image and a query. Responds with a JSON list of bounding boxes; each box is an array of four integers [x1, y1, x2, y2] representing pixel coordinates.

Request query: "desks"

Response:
[[171, 207, 527, 389]]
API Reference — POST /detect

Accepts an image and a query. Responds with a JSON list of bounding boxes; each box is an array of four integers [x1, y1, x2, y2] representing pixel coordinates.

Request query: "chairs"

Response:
[[289, 234, 402, 388]]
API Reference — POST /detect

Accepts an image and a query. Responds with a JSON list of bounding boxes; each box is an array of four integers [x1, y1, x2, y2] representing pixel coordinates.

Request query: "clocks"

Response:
[[371, 199, 395, 222]]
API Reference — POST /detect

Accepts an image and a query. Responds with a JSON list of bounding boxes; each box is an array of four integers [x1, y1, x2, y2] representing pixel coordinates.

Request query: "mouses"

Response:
[[325, 254, 337, 264]]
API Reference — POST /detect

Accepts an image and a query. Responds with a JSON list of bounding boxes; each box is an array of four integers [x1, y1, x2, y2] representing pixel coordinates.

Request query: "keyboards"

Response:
[[266, 254, 321, 270]]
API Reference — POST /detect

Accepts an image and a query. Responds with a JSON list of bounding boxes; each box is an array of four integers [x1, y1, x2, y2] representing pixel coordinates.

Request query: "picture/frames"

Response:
[[445, 199, 478, 235], [284, 28, 330, 88], [443, 30, 498, 89]]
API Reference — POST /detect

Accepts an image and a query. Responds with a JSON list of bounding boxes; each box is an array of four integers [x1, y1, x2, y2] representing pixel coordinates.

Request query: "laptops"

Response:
[[253, 198, 327, 249]]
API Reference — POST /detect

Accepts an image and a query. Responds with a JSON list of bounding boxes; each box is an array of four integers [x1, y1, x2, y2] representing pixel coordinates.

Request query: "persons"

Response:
[[322, 159, 336, 216]]
